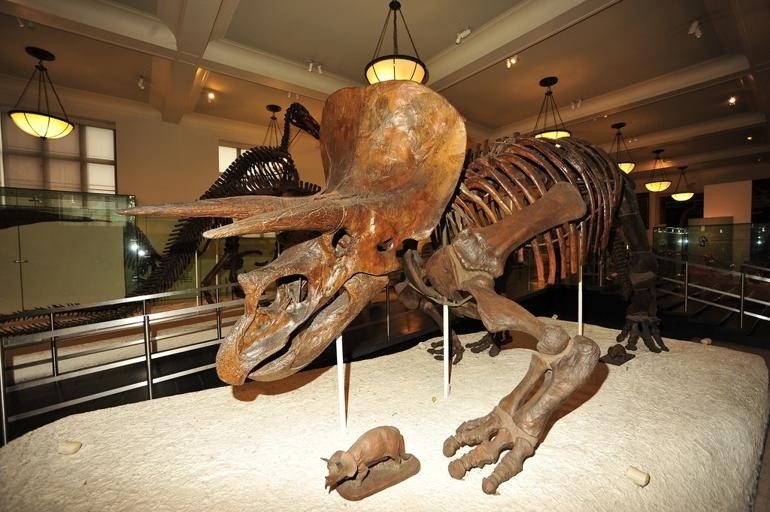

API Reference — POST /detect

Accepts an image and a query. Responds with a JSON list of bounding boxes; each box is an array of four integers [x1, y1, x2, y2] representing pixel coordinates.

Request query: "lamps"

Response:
[[364, 1, 431, 85], [525, 75, 697, 203], [8, 45, 76, 142]]
